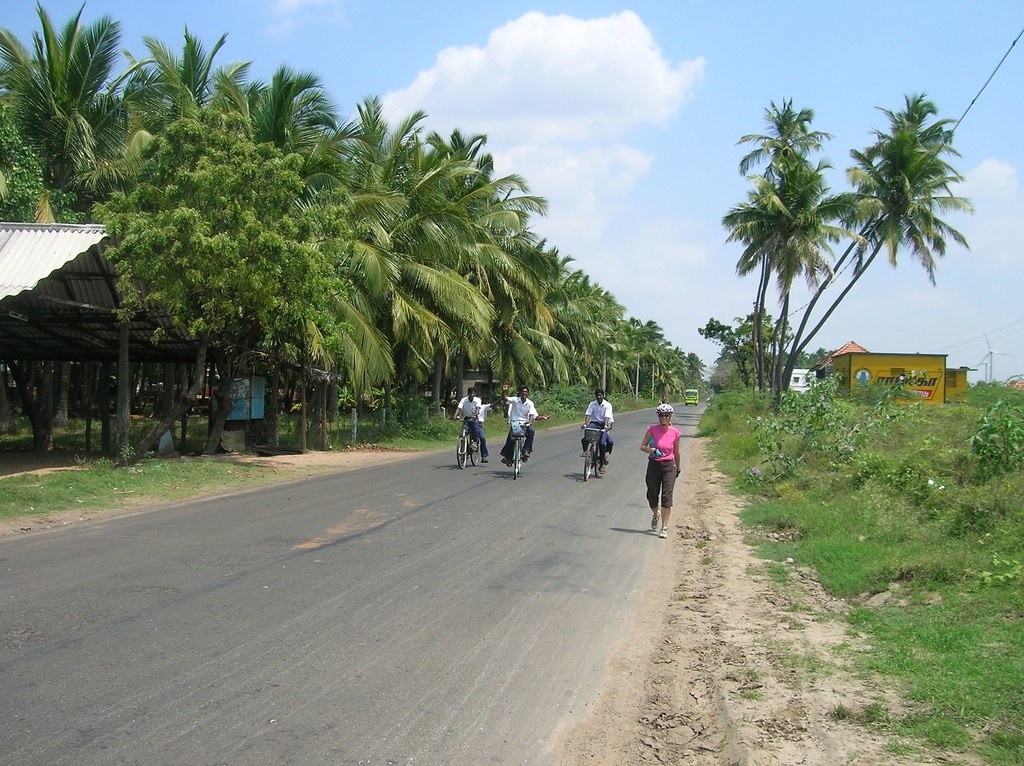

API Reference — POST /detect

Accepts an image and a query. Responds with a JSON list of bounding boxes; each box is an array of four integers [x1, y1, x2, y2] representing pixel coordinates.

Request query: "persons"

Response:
[[580, 390, 614, 474], [661, 396, 665, 404], [452, 381, 551, 465], [640, 404, 681, 538]]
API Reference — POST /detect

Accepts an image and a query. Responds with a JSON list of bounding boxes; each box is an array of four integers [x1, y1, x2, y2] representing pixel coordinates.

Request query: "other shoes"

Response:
[[605, 457, 609, 465], [482, 456, 488, 463], [469, 442, 478, 448], [523, 449, 530, 462], [581, 452, 586, 457], [502, 457, 512, 467], [599, 464, 606, 473]]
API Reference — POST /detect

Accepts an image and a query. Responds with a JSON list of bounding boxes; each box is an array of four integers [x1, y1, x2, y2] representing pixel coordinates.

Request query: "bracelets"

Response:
[[454, 411, 458, 414], [654, 448, 657, 453], [604, 425, 608, 428], [677, 469, 681, 473]]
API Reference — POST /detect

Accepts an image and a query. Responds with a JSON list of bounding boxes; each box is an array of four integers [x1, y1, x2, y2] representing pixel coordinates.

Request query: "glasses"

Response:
[[659, 414, 671, 417]]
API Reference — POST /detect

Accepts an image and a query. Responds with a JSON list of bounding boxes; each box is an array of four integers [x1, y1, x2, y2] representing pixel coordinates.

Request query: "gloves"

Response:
[[653, 448, 663, 457], [676, 471, 681, 478]]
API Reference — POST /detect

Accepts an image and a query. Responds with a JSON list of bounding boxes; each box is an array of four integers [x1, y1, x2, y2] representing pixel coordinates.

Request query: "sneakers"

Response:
[[659, 527, 668, 538], [651, 510, 661, 529]]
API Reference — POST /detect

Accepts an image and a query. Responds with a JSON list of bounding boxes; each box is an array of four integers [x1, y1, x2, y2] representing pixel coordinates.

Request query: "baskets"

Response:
[[585, 429, 601, 443], [511, 426, 527, 437]]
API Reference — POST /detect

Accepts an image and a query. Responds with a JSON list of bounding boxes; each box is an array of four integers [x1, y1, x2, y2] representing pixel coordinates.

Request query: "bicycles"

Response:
[[453, 413, 481, 469], [581, 425, 609, 481], [506, 421, 533, 480]]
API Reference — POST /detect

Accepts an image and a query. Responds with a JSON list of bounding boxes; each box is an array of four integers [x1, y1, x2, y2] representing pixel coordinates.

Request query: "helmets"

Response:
[[656, 404, 673, 414]]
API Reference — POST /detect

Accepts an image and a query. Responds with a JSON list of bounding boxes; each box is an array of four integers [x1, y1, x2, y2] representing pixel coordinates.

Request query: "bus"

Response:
[[684, 389, 699, 406]]
